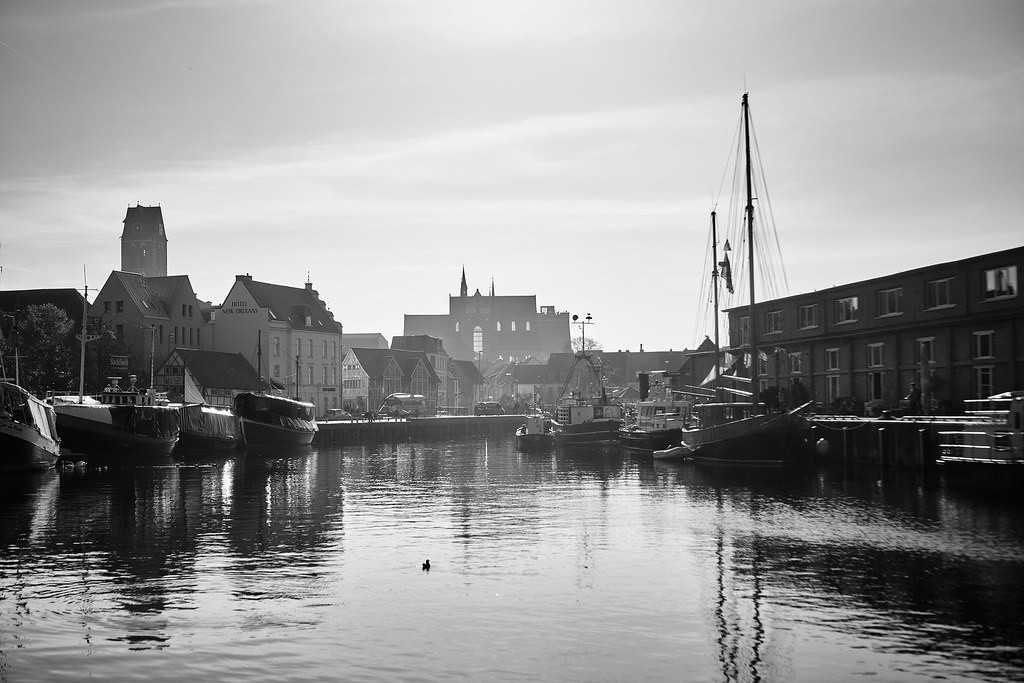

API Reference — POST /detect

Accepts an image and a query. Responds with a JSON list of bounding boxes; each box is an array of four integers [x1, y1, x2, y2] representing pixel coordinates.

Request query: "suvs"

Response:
[[474, 402, 505, 416]]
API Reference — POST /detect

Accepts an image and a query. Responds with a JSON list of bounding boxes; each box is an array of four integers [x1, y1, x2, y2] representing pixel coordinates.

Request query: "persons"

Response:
[[908, 382, 919, 416], [368, 411, 373, 423]]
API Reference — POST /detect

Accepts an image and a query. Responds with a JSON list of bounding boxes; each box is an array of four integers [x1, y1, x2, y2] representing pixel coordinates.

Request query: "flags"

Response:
[[721, 252, 735, 294]]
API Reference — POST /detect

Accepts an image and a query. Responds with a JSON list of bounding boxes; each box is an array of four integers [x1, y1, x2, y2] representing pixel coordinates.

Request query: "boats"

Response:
[[0, 348, 61, 473], [230, 328, 320, 446], [652, 447, 688, 460], [42, 262, 182, 461], [514, 393, 554, 453], [551, 313, 625, 444], [100, 375, 244, 454], [619, 380, 700, 449]]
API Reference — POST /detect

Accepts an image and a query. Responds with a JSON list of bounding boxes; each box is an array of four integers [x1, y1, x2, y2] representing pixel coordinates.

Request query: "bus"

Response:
[[385, 393, 425, 418]]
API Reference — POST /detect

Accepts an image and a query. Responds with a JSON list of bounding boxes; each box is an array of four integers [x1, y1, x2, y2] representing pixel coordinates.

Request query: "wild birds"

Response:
[[422, 560, 430, 567]]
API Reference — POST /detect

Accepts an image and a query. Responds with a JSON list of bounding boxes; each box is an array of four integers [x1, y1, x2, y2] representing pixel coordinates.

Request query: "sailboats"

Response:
[[681, 90, 819, 469]]
